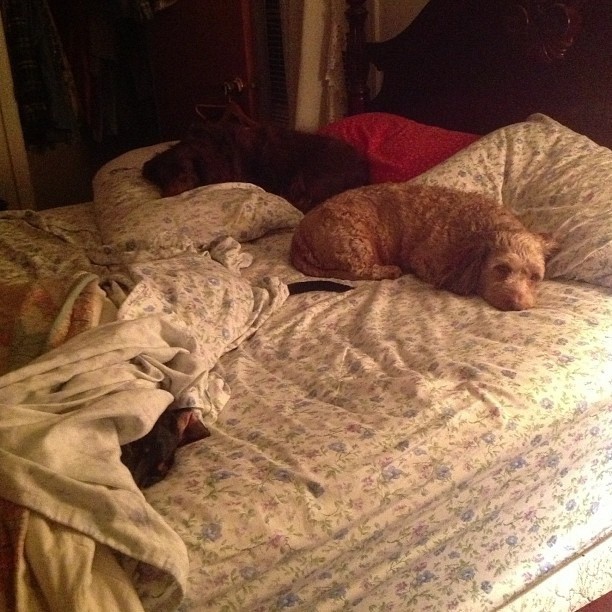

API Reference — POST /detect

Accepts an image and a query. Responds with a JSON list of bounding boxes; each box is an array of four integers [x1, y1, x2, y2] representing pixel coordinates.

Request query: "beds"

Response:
[[0, 0, 611, 609]]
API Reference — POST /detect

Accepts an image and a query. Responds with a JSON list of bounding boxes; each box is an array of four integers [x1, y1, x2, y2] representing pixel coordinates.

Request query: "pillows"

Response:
[[316, 109, 486, 188]]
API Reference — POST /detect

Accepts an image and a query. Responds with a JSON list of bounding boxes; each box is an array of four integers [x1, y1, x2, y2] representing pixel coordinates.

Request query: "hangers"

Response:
[[193, 76, 250, 129]]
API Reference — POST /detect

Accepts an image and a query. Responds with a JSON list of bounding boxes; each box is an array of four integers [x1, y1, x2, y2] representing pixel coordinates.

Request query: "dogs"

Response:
[[120, 280, 357, 490], [288, 182, 566, 311], [140, 119, 374, 215]]
